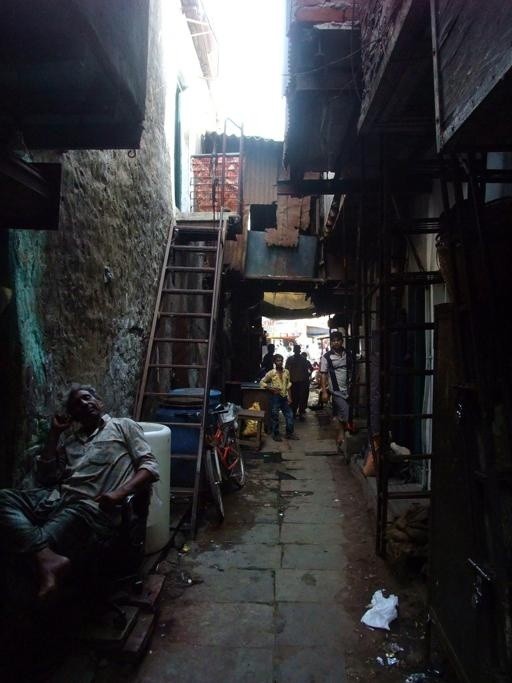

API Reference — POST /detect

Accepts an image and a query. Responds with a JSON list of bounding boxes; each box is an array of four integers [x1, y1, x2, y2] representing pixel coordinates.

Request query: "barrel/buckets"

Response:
[[135, 422, 171, 555], [168, 388, 222, 455]]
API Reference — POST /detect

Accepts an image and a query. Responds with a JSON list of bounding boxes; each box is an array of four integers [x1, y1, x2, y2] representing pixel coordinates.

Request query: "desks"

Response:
[[235, 409, 266, 451]]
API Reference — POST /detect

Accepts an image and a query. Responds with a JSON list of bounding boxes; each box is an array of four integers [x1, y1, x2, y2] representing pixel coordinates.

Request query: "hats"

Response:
[[330, 331, 343, 340], [294, 344, 301, 349]]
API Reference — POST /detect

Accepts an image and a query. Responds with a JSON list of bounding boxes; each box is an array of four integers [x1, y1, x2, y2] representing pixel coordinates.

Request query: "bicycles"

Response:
[[175, 403, 247, 522]]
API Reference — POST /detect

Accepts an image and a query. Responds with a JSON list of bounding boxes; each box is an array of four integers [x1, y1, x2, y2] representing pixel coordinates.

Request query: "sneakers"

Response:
[[272, 433, 283, 443], [284, 432, 301, 441]]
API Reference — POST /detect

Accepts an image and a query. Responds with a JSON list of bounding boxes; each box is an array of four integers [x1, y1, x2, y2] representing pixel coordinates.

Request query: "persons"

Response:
[[260, 353, 300, 441], [0, 379, 162, 634], [262, 343, 275, 369], [301, 352, 312, 413], [321, 331, 351, 444], [284, 344, 307, 419]]
